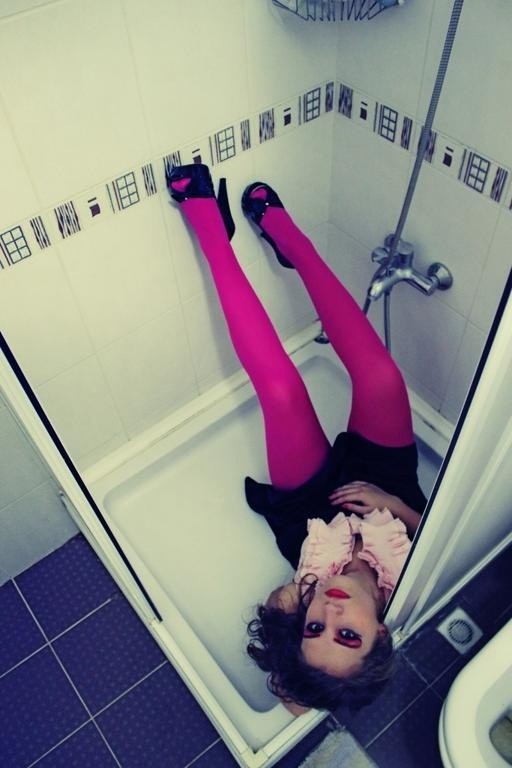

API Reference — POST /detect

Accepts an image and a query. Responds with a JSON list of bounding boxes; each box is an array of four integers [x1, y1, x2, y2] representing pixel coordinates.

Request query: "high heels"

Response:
[[168, 165, 235, 242]]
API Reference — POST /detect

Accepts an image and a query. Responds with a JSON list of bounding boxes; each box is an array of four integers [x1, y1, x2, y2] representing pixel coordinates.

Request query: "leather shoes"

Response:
[[241, 182, 295, 268]]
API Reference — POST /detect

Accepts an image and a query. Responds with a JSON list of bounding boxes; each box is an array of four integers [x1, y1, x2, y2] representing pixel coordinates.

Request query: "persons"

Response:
[[166, 163, 428, 717]]
